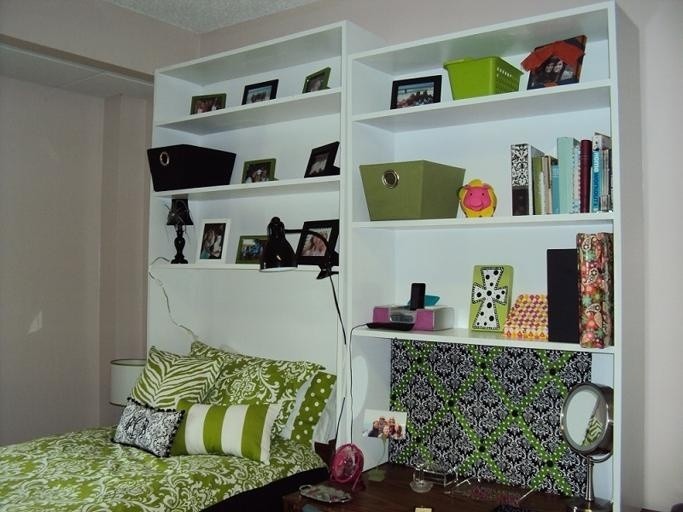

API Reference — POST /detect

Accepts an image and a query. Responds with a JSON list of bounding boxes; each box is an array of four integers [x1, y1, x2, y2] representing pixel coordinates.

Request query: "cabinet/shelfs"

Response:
[[146, 19, 389, 444], [346, 0, 641, 512]]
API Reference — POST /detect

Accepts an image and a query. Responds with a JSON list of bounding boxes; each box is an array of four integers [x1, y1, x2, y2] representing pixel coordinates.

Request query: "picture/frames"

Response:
[[190, 66, 331, 115], [241, 141, 340, 184], [526, 34, 587, 90], [194, 218, 339, 265], [390, 75, 442, 109]]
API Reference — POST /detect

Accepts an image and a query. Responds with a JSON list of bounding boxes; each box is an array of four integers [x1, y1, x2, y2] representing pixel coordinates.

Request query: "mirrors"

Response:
[[559, 381, 614, 512]]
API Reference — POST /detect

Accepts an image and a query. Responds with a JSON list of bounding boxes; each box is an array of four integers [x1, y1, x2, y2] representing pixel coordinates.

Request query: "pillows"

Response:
[[110, 340, 338, 467]]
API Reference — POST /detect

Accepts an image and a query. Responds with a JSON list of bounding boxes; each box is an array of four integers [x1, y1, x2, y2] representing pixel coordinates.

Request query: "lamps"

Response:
[[109, 359, 146, 407], [166, 199, 194, 264]]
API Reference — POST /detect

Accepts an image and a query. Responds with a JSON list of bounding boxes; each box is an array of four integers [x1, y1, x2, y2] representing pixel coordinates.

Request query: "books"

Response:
[[508, 130, 611, 216]]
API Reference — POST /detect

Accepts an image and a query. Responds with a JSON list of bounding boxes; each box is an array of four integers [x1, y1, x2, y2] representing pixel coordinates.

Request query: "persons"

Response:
[[259, 167, 269, 184], [396, 88, 435, 107], [245, 170, 257, 183], [304, 231, 327, 256], [362, 414, 403, 441], [547, 57, 575, 82], [527, 58, 553, 88], [201, 228, 221, 259]]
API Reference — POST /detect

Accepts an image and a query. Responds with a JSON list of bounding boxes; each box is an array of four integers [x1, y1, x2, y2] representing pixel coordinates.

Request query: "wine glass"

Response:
[[408, 449, 434, 492]]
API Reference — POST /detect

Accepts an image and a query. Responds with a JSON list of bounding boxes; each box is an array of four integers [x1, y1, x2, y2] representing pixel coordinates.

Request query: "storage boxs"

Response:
[[358, 159, 466, 221], [147, 143, 237, 192]]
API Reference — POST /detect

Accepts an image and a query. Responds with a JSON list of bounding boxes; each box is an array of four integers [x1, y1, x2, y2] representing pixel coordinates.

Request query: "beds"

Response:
[[0, 421, 334, 511]]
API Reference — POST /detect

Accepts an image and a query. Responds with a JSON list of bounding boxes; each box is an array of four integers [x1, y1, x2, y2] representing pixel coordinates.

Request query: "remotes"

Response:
[[365, 322, 414, 331]]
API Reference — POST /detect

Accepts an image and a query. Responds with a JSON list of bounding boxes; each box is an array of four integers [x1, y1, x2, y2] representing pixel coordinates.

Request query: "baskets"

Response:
[[441, 55, 525, 102]]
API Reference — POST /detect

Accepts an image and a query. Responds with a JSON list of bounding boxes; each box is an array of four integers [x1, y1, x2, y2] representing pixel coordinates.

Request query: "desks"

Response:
[[282, 460, 613, 512]]
